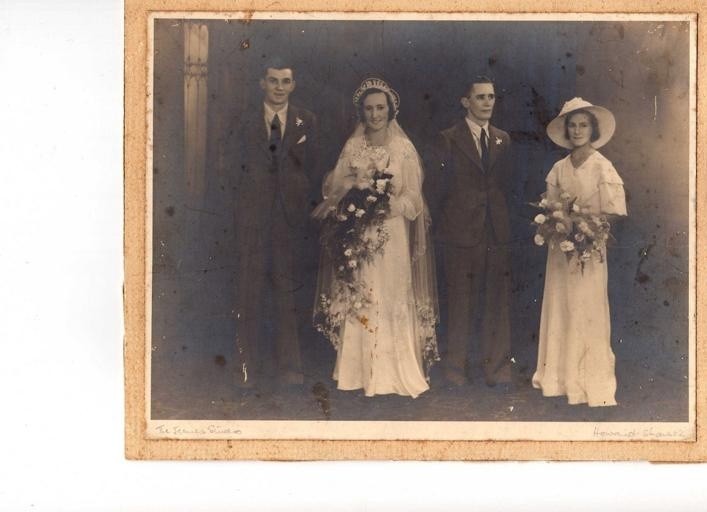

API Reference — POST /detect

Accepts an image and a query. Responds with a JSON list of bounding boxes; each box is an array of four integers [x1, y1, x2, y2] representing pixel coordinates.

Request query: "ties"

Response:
[[481, 128, 489, 161], [270, 113, 282, 157]]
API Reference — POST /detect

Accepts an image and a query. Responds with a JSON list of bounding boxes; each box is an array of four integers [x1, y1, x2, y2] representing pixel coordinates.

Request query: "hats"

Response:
[[545, 96, 615, 149]]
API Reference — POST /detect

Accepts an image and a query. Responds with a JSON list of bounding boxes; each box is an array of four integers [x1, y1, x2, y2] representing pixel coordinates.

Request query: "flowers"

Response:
[[526, 188, 618, 277], [310, 158, 395, 351]]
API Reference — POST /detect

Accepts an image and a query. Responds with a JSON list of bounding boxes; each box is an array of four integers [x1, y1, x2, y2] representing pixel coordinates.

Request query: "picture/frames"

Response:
[[124, 0, 707, 463]]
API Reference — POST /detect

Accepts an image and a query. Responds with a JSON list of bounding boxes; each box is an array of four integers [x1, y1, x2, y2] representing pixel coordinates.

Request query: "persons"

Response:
[[422, 74, 516, 389], [317, 80, 425, 398], [230, 55, 321, 392], [533, 95, 628, 407]]
